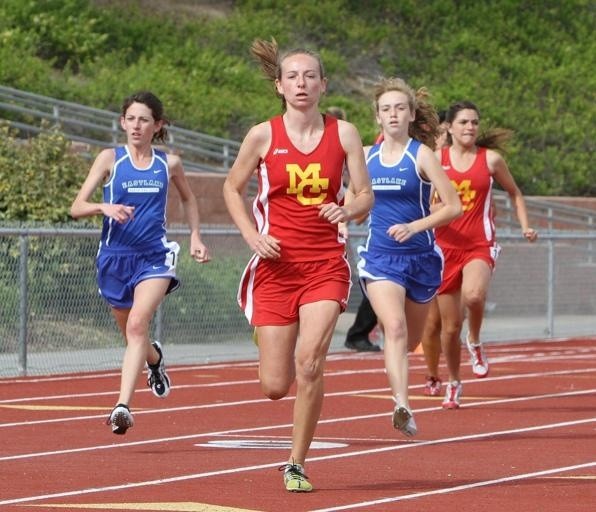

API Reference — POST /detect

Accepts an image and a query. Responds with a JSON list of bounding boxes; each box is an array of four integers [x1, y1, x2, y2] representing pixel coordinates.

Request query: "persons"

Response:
[[322, 105, 351, 124], [432, 101, 539, 411], [347, 296, 381, 352], [345, 78, 464, 437], [435, 122, 449, 148], [71, 90, 211, 433], [422, 296, 443, 395], [222, 36, 376, 492]]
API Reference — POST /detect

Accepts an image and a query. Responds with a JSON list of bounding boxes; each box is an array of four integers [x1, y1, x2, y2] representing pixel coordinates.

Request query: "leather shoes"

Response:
[[345, 336, 380, 352]]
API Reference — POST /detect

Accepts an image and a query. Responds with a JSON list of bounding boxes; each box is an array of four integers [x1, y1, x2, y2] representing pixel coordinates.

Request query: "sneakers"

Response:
[[145, 341, 170, 398], [278, 456, 312, 493], [392, 403, 417, 437], [442, 380, 462, 409], [423, 375, 442, 396], [466, 330, 490, 378], [107, 404, 133, 434]]
